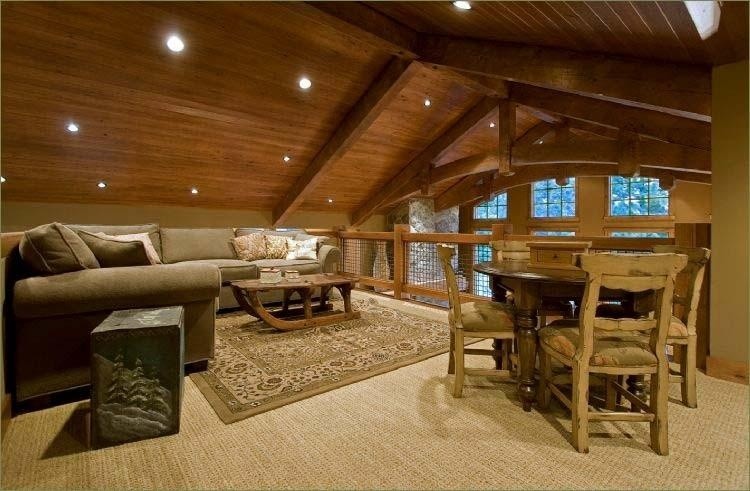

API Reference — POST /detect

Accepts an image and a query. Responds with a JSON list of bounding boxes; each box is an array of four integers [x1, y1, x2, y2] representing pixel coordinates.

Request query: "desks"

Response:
[[472, 260, 654, 414]]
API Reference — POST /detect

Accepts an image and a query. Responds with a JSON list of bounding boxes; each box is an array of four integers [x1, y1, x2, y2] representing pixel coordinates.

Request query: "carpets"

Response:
[[188, 297, 485, 425]]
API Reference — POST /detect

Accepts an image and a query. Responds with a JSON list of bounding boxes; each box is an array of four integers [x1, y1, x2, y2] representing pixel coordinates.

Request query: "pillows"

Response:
[[162, 227, 329, 265], [18, 221, 161, 276]]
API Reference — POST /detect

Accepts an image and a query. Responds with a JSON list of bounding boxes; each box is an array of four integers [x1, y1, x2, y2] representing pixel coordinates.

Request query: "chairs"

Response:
[[437, 244, 536, 401], [536, 250, 690, 455], [485, 240, 594, 372], [618, 244, 713, 408]]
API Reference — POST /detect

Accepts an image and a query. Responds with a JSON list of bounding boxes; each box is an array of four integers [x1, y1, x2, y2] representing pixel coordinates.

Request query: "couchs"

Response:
[[9, 223, 343, 418]]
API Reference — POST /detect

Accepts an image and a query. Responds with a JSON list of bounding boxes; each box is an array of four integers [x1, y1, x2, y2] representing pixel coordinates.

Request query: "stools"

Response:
[[90, 305, 185, 449]]
